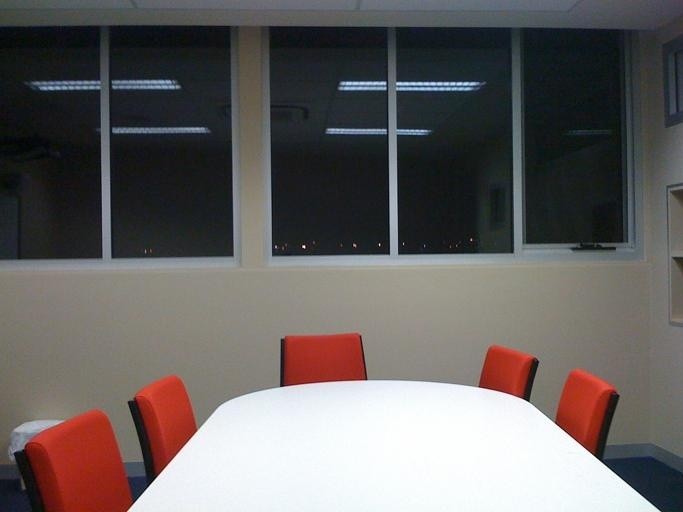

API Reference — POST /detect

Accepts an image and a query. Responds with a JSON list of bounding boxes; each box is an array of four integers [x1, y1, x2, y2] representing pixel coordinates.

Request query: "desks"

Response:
[[126, 380, 661, 512]]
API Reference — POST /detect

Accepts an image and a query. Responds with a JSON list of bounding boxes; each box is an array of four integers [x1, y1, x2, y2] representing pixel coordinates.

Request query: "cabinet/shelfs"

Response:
[[666, 184, 683, 327]]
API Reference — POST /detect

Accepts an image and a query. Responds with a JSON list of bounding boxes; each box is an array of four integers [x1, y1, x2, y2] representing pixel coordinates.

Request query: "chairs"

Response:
[[479, 344, 540, 402], [555, 367, 620, 460], [127, 375, 197, 487], [14, 409, 134, 512], [279, 333, 368, 387]]
